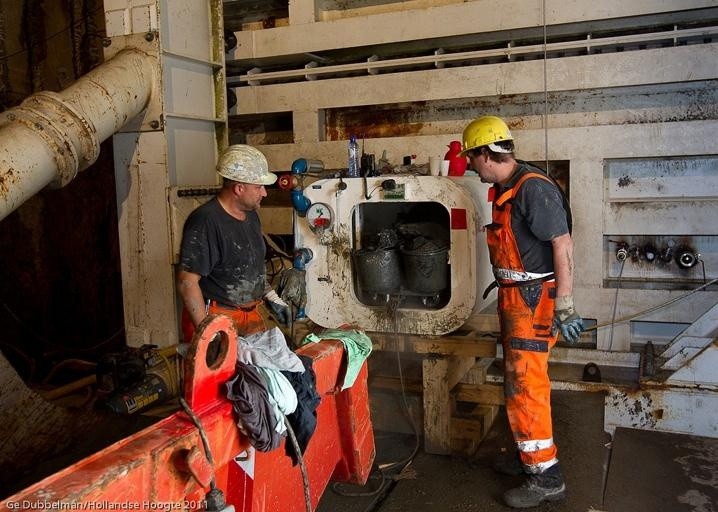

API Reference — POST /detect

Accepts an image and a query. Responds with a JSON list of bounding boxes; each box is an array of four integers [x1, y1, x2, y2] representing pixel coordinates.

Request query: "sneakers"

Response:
[[504, 477, 568, 508]]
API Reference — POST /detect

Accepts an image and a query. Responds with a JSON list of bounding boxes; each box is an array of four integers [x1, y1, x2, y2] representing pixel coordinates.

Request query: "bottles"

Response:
[[347, 135, 360, 178], [444, 141, 467, 176]]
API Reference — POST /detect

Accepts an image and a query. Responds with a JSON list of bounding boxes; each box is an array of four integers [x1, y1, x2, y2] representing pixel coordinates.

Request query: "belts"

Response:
[[204, 299, 256, 312], [482, 273, 556, 300]]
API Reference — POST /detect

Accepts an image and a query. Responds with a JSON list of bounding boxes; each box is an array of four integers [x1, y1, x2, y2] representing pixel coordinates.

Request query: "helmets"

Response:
[[457, 116, 514, 157], [215, 144, 277, 185]]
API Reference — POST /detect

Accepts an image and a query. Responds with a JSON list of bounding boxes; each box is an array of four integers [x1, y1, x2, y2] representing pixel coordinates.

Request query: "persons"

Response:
[[175, 143, 292, 343], [456, 116, 585, 509]]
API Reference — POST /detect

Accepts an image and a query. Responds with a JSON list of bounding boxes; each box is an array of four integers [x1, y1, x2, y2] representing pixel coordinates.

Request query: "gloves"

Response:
[[551, 296, 586, 344], [263, 289, 293, 329]]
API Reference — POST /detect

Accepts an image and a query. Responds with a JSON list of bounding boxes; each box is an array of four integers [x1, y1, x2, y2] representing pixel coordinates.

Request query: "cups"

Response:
[[429, 154, 451, 177]]
